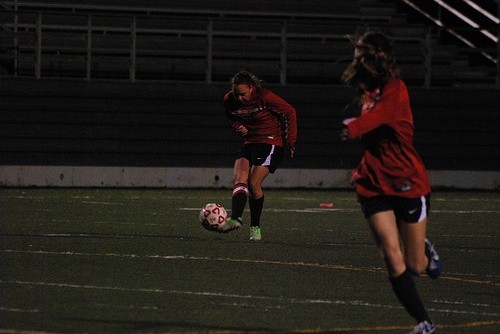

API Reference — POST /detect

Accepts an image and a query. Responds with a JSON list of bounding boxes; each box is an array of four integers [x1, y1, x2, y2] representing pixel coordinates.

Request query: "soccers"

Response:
[[198, 202, 228, 232]]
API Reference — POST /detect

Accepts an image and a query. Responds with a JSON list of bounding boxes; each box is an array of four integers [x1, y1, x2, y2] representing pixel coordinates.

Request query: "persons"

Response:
[[339, 29, 442, 334], [215, 70, 298, 243]]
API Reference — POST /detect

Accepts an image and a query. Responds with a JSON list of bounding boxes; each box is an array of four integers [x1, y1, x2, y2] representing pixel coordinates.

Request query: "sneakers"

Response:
[[249, 226, 261, 241], [409, 320, 437, 334], [425, 236, 443, 280], [219, 217, 243, 234]]
